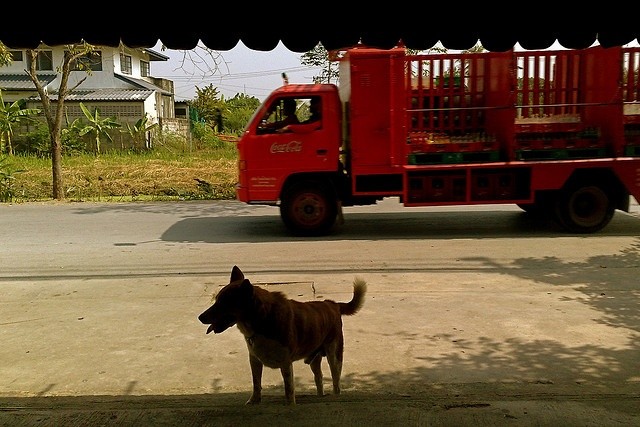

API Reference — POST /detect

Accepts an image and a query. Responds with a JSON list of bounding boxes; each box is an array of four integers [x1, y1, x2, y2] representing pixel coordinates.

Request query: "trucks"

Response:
[[236, 43, 640, 234]]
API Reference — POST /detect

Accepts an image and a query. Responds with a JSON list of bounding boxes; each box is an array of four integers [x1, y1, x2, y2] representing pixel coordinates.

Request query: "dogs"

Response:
[[197, 264, 367, 409]]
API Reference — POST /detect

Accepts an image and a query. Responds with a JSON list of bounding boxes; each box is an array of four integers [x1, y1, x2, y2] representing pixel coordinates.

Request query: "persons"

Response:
[[263, 99, 299, 128], [276, 95, 320, 132]]
